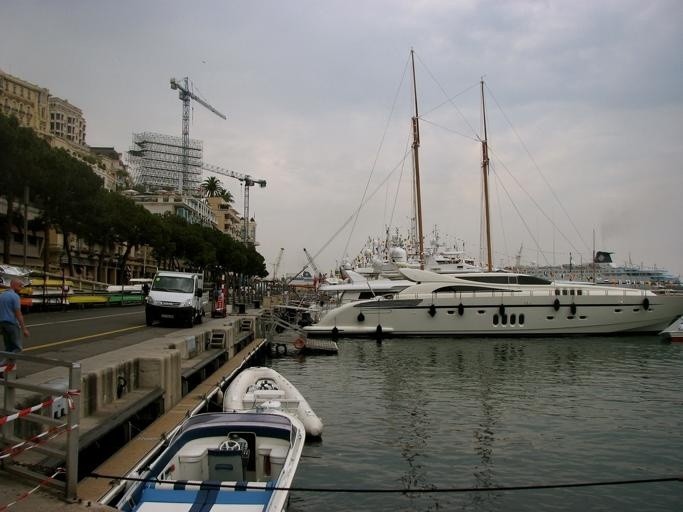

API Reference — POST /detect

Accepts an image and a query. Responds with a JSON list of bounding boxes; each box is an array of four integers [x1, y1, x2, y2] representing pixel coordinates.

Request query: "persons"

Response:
[[0, 278, 30, 378]]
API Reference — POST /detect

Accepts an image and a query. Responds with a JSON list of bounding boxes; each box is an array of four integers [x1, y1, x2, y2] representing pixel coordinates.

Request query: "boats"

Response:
[[108, 407, 307, 512], [224, 365, 320, 438]]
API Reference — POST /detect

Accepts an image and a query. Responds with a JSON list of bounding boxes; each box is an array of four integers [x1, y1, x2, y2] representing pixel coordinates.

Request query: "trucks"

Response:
[[142, 268, 210, 324]]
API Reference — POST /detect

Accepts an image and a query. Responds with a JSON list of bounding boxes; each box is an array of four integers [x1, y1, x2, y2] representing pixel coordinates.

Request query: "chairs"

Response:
[[252, 390, 284, 410], [208, 450, 244, 482]]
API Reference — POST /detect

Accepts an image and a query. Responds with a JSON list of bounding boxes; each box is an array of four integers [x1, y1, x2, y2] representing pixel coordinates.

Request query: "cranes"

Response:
[[178, 154, 266, 249], [167, 74, 228, 198]]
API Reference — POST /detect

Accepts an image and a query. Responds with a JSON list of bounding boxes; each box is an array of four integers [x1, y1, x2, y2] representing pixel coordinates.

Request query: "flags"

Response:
[[594, 251, 614, 263]]
